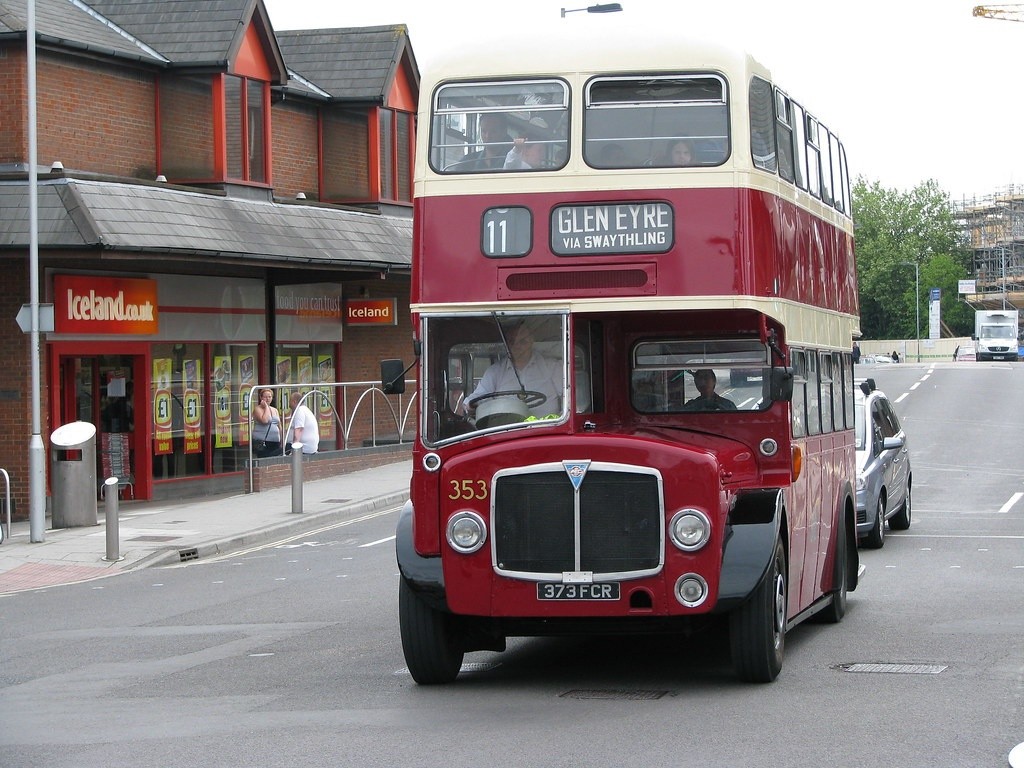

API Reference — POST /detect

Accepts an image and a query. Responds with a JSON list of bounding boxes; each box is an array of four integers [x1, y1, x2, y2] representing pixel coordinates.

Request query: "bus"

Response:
[[381, 40, 865, 684]]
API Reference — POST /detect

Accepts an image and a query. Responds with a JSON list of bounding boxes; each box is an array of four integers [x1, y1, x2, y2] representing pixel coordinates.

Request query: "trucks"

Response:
[[971, 310, 1021, 362]]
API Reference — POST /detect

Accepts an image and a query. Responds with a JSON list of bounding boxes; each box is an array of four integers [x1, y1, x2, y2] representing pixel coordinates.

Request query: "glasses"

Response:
[[507, 339, 532, 345], [695, 374, 713, 380]]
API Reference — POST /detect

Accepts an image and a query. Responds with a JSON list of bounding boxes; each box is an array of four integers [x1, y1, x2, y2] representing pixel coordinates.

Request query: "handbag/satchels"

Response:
[[248, 438, 266, 454]]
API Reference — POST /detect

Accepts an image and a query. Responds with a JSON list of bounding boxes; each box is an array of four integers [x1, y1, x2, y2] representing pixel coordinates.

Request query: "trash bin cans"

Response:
[[49, 420, 97, 529]]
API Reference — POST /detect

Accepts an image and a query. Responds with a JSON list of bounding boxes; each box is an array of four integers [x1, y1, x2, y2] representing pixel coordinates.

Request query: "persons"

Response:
[[892, 351, 899, 363], [681, 370, 737, 410], [462, 321, 563, 420], [253, 389, 282, 454], [252, 393, 319, 459], [853, 342, 861, 364], [455, 112, 695, 170]]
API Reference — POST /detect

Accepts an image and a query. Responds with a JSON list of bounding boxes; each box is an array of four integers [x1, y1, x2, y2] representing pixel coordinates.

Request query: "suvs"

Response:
[[851, 377, 912, 548]]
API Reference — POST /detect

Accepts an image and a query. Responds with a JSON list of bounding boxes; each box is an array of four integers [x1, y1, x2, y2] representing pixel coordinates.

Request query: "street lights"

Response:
[[901, 262, 920, 362]]
